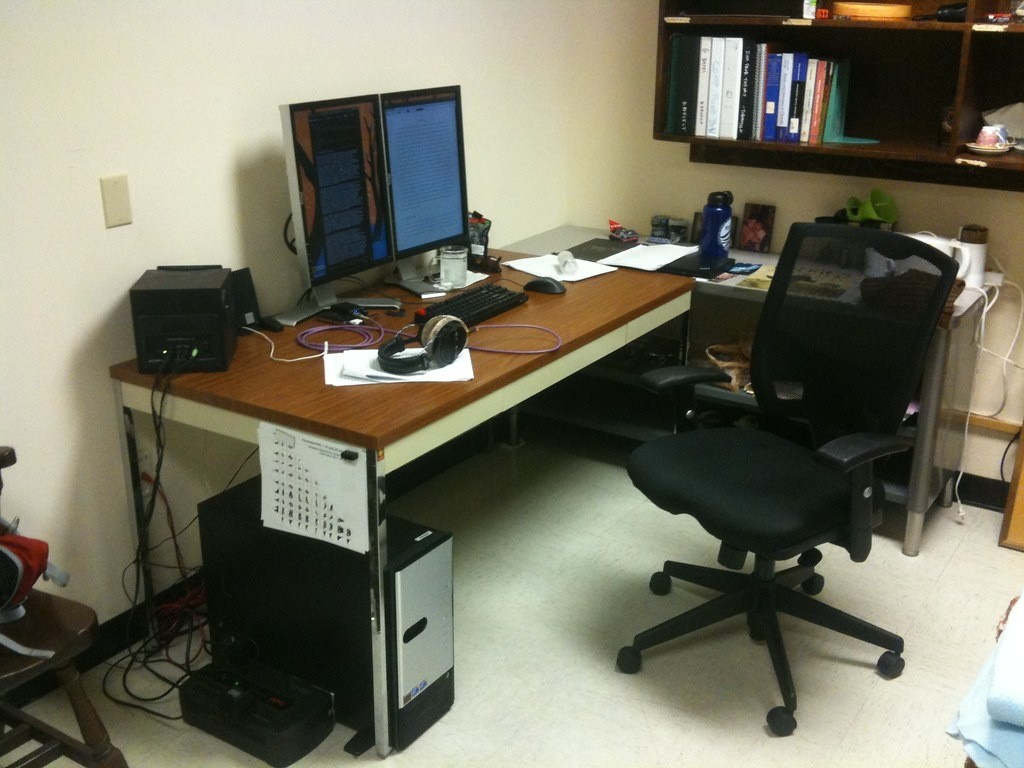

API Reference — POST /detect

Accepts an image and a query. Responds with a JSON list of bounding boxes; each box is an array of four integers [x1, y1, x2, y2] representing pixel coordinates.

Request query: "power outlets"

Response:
[[101, 173, 132, 230]]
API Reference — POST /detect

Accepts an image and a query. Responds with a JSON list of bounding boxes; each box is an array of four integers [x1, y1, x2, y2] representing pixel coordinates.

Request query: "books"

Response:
[[659, 32, 836, 146]]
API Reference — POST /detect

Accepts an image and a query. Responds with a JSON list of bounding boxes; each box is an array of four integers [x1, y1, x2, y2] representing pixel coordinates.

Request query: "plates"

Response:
[[965, 142, 1017, 155]]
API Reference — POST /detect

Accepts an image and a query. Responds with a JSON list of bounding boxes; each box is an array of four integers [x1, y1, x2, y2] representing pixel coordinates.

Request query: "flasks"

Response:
[[959, 224, 987, 286]]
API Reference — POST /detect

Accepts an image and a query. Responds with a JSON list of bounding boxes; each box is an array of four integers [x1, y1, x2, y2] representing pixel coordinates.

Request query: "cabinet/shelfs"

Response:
[[652, 0, 1023, 195]]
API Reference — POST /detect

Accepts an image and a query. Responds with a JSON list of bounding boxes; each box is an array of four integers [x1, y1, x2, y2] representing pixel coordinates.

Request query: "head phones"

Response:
[[377, 315, 469, 375]]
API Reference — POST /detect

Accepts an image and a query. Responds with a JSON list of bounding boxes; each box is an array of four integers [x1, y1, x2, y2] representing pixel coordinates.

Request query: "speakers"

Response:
[[227, 267, 265, 338]]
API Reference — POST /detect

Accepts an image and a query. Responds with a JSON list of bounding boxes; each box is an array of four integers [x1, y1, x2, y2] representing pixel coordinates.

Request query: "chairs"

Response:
[[616, 222, 960, 738], [0, 446, 132, 768]]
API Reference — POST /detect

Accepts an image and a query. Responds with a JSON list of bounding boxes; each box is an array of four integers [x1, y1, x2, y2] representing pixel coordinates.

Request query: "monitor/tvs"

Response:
[[278, 84, 471, 298]]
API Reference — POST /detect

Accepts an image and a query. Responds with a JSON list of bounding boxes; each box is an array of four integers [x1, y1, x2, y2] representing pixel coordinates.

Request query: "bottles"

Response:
[[699, 191, 733, 258]]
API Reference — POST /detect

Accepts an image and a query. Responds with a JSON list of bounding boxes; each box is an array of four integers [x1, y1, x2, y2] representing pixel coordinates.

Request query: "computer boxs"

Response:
[[197, 470, 456, 753]]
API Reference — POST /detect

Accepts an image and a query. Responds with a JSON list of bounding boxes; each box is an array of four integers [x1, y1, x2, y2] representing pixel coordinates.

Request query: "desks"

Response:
[[110, 247, 701, 760], [498, 220, 991, 559]]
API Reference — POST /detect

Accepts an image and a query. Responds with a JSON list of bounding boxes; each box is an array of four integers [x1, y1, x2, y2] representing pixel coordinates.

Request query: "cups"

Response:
[[977, 125, 1005, 149], [994, 124, 1017, 143], [903, 233, 971, 280], [427, 245, 468, 287]]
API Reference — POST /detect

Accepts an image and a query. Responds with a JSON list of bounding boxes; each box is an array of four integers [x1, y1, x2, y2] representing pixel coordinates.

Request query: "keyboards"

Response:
[[415, 282, 529, 327]]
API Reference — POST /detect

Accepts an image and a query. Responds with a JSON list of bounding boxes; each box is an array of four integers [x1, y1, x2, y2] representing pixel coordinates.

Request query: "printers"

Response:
[[129, 263, 238, 375]]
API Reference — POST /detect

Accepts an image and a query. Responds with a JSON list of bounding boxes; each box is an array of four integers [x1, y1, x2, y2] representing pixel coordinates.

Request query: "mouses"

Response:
[[523, 276, 566, 295]]
